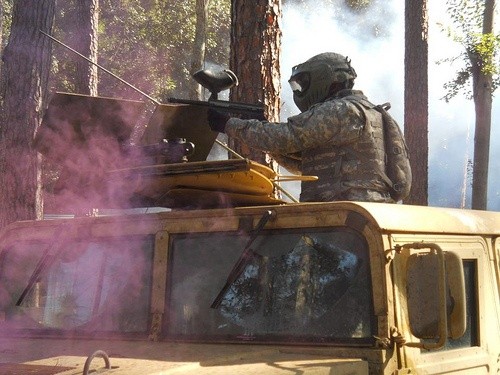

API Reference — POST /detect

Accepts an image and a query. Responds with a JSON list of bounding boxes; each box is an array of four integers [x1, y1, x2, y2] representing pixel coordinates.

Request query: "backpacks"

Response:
[[340, 97, 413, 199]]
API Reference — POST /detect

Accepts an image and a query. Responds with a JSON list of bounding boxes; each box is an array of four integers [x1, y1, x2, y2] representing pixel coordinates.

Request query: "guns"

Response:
[[165, 67, 270, 122]]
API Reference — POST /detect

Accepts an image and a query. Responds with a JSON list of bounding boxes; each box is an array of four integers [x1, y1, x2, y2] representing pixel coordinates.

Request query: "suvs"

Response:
[[0, 30, 500, 375]]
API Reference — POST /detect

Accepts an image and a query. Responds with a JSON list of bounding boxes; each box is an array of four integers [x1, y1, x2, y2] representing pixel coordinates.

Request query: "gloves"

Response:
[[207, 108, 230, 131]]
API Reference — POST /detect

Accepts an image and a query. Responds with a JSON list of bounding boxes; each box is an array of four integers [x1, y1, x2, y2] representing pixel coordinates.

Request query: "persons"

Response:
[[207, 52, 396, 204]]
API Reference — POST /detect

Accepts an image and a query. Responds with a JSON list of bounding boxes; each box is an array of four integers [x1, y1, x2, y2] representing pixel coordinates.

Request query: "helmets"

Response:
[[288, 52, 356, 113]]
